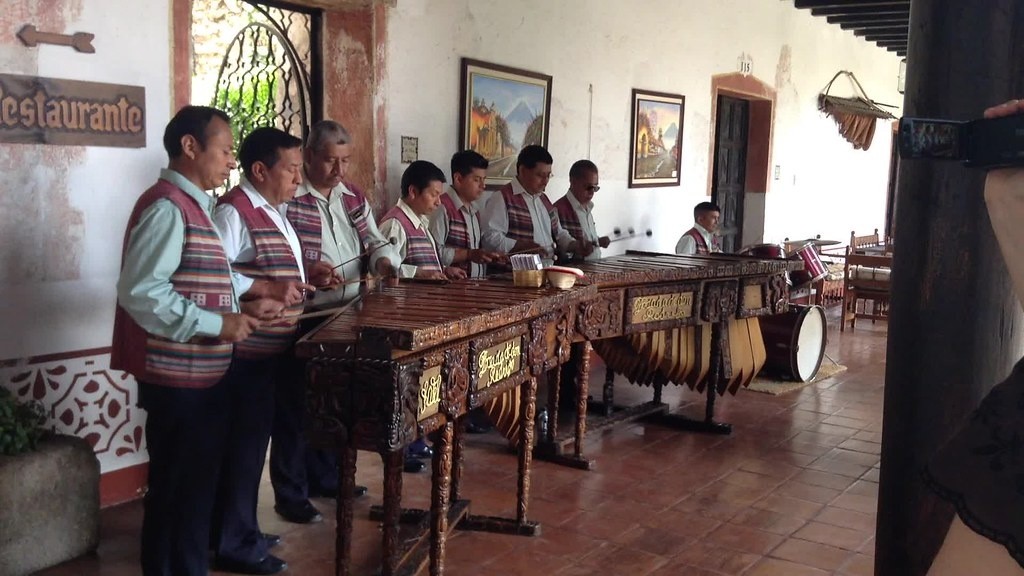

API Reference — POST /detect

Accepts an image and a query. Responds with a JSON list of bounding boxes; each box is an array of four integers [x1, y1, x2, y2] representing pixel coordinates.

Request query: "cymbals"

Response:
[[783, 239, 842, 246]]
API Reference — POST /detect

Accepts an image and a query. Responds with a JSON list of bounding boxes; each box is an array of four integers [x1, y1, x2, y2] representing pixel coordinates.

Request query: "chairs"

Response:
[[785, 228, 892, 333]]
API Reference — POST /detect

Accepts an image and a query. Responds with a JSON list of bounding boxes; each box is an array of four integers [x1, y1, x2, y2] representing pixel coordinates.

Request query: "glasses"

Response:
[[526, 169, 554, 179], [578, 179, 601, 192]]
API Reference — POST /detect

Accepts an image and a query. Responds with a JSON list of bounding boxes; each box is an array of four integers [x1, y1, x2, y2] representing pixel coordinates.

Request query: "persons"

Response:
[[553, 159, 611, 262], [377, 160, 468, 472], [675, 202, 724, 254], [919, 99, 1024, 576], [110, 105, 284, 576], [425, 150, 510, 433], [210, 128, 338, 576], [480, 144, 594, 276], [270, 120, 401, 523]]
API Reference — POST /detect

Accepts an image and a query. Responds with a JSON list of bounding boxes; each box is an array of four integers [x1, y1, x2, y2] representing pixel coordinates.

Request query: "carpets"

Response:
[[739, 364, 848, 395]]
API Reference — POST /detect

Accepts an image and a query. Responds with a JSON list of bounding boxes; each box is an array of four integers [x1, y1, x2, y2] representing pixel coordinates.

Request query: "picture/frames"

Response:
[[628, 88, 685, 189], [458, 57, 553, 190]]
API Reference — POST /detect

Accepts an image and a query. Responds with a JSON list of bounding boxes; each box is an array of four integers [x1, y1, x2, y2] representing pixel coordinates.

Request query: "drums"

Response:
[[787, 241, 829, 290], [760, 302, 828, 383], [735, 243, 782, 258]]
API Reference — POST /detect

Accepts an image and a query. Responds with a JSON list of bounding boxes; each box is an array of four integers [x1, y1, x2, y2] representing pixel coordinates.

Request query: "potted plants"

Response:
[[0, 388, 103, 576]]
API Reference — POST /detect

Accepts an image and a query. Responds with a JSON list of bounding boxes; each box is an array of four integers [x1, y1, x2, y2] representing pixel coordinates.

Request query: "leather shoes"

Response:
[[316, 481, 368, 498], [262, 534, 281, 546], [214, 555, 288, 574], [275, 499, 323, 525], [404, 459, 427, 473], [409, 446, 435, 459]]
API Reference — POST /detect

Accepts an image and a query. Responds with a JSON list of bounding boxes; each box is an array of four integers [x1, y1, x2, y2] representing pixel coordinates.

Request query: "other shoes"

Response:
[[467, 421, 491, 434]]
